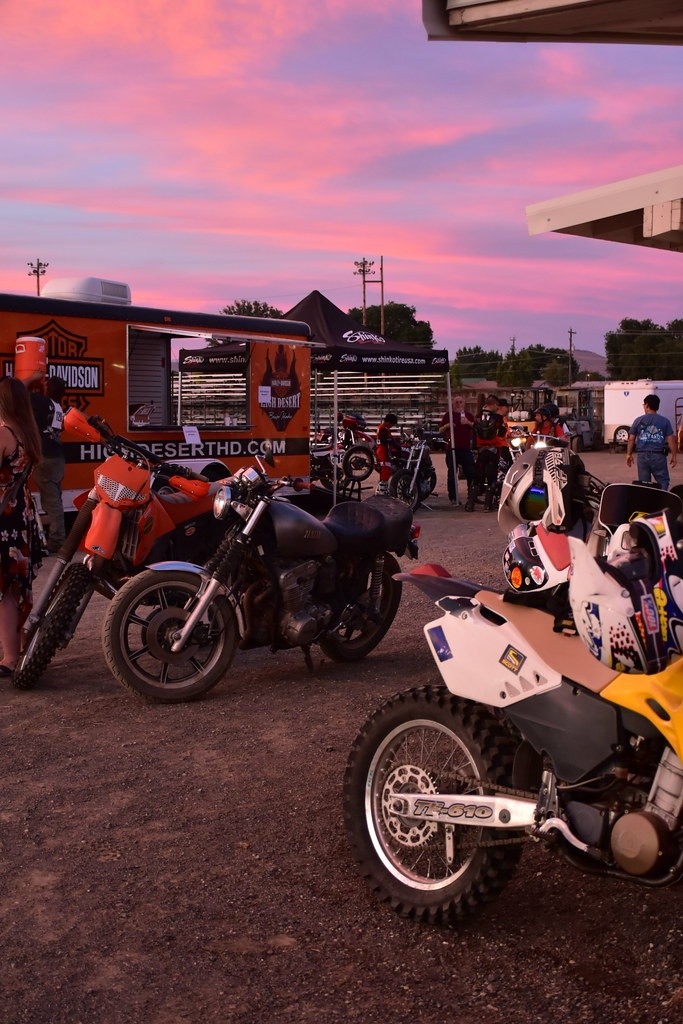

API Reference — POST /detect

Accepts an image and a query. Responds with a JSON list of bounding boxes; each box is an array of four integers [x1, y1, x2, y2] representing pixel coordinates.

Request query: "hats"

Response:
[[386, 414, 399, 428], [498, 399, 512, 406]]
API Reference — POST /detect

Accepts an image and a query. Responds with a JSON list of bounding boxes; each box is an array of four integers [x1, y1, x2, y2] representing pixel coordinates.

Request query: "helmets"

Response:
[[498, 447, 586, 535], [567, 508, 683, 674], [534, 404, 559, 418]]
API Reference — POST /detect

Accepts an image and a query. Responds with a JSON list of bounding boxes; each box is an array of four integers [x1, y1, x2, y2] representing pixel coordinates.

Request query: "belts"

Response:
[[638, 451, 665, 454]]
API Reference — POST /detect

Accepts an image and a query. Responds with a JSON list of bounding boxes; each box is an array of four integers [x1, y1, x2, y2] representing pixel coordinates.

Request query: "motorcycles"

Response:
[[310, 409, 375, 502], [339, 557, 682, 931], [102, 447, 421, 704], [501, 481, 682, 677], [11, 416, 242, 693], [434, 425, 683, 519], [387, 423, 441, 511]]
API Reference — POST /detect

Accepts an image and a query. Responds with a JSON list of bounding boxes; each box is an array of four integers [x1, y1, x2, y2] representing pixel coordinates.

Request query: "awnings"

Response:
[[128, 324, 326, 359]]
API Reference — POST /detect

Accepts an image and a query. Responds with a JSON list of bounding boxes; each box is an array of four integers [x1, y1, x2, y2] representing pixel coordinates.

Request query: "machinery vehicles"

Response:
[[506, 385, 556, 433]]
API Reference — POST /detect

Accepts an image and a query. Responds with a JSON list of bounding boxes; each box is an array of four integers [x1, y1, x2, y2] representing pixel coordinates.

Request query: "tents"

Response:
[[278, 290, 459, 511]]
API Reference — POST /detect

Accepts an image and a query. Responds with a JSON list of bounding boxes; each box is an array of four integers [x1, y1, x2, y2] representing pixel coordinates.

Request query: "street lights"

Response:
[[353, 257, 376, 330], [27, 257, 50, 298]]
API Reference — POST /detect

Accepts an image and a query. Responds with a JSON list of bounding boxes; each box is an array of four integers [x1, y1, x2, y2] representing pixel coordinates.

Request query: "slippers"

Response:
[[0, 665, 14, 678]]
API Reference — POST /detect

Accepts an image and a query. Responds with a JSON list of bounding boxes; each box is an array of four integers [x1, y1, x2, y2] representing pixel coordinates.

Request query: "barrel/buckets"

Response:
[[14, 337, 47, 390]]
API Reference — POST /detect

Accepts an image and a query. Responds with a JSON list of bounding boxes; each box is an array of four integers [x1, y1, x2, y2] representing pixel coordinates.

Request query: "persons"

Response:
[[374, 414, 398, 495], [0, 377, 44, 678], [439, 394, 514, 513], [526, 403, 570, 452], [626, 394, 677, 491], [22, 367, 65, 554]]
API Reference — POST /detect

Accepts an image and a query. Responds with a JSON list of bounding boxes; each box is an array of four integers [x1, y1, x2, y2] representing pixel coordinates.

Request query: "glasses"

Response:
[[519, 451, 549, 521]]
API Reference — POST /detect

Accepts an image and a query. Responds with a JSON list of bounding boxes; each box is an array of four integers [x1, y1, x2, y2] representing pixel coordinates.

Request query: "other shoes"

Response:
[[450, 499, 462, 505], [46, 545, 60, 553], [464, 504, 473, 512], [484, 506, 495, 513]]
